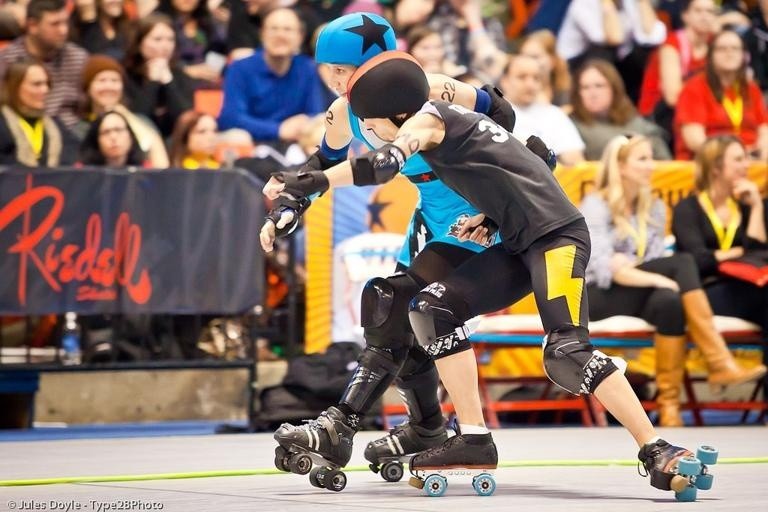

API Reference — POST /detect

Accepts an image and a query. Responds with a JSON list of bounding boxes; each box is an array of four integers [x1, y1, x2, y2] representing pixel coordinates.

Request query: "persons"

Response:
[[0, 0, 768, 476]]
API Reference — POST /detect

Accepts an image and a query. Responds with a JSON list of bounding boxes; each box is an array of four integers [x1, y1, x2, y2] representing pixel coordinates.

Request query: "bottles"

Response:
[[60, 309, 84, 367]]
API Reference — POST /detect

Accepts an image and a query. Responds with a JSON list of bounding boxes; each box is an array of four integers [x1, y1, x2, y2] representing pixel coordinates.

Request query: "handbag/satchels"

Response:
[[718, 250, 768, 286]]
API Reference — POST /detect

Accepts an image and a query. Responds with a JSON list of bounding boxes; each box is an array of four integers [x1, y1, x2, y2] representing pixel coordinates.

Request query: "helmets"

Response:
[[348, 50, 429, 118], [316, 12, 396, 67]]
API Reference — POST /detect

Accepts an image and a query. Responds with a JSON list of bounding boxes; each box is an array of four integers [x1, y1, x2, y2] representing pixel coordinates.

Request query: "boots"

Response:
[[682, 287, 766, 395], [656, 334, 688, 426]]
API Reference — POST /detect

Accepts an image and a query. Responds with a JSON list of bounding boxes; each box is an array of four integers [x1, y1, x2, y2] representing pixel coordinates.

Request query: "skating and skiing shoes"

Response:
[[274, 406, 358, 491], [638, 439, 718, 502], [364, 417, 498, 496]]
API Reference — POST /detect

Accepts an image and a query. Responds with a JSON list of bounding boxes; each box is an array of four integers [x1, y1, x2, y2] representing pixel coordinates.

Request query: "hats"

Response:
[[80, 54, 128, 96]]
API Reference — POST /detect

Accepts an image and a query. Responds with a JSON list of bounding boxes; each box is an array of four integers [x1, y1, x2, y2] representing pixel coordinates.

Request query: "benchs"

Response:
[[376, 310, 764, 429]]
